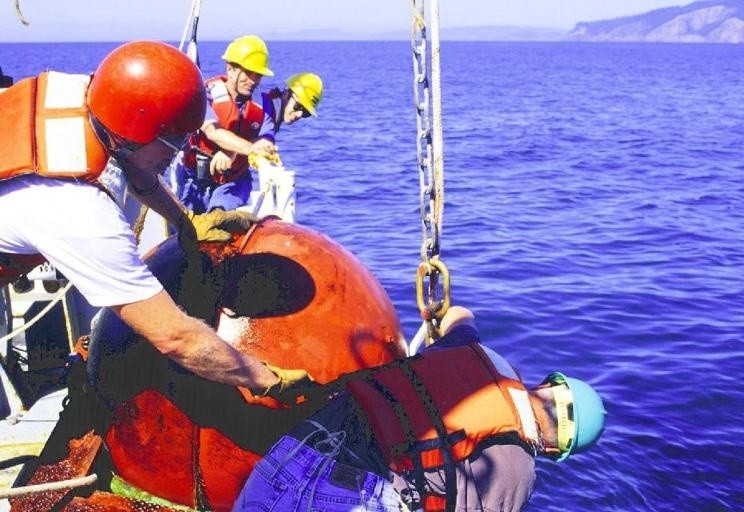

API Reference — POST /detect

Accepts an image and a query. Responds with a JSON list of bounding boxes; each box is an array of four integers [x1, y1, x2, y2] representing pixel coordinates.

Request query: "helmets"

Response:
[[86, 39, 207, 146], [283, 72, 324, 118], [543, 370, 609, 464], [220, 34, 275, 78]]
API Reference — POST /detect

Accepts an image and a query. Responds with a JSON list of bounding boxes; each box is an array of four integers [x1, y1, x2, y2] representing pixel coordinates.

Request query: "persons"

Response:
[[258, 72, 327, 132], [0, 40, 325, 420], [167, 33, 278, 236], [233, 306, 607, 512]]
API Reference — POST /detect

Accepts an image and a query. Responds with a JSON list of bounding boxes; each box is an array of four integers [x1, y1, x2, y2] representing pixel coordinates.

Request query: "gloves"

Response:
[[249, 360, 314, 399], [178, 206, 263, 243]]
